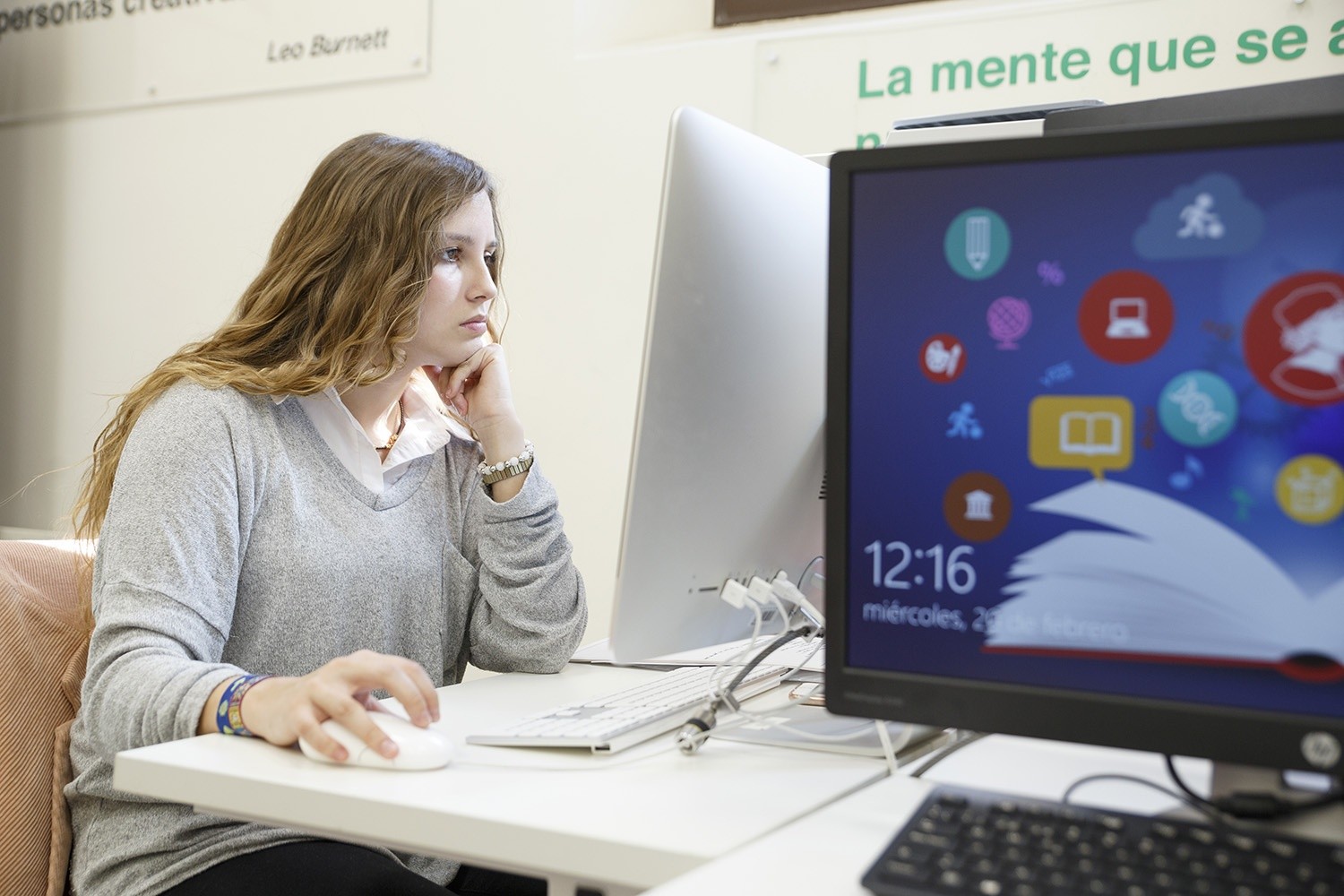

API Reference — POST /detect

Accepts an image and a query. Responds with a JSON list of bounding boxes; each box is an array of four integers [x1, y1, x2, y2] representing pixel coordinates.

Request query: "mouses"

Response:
[[297, 712, 461, 772]]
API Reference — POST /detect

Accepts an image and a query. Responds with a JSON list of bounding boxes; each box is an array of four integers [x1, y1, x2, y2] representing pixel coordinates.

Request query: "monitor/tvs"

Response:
[[825, 115, 1344, 834], [613, 104, 944, 760]]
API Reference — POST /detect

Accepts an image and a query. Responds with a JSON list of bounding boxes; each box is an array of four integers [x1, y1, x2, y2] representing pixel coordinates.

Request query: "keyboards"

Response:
[[859, 784, 1344, 896], [468, 666, 787, 750]]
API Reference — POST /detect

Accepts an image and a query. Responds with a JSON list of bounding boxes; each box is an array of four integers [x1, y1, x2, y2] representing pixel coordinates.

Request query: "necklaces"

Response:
[[374, 400, 404, 450]]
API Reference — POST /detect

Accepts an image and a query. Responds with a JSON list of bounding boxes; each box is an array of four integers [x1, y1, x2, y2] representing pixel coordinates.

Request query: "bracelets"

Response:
[[216, 675, 255, 735], [479, 441, 535, 485], [228, 675, 271, 737]]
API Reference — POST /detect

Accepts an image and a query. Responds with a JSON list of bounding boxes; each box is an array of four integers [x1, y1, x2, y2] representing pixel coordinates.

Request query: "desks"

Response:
[[110, 634, 1344, 896]]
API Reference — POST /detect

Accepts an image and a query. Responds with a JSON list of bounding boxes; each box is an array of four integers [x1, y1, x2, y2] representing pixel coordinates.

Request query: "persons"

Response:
[[63, 133, 589, 896]]
